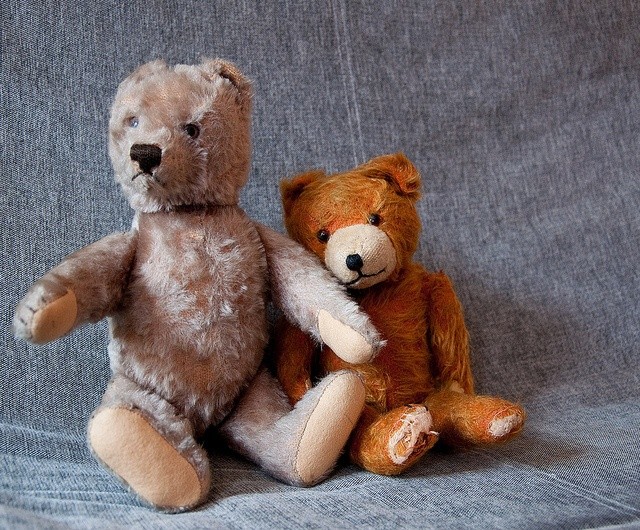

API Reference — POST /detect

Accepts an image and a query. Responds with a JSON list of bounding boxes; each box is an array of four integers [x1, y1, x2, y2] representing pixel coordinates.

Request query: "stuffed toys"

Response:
[[12, 58, 387, 515], [279, 153, 528, 477]]
